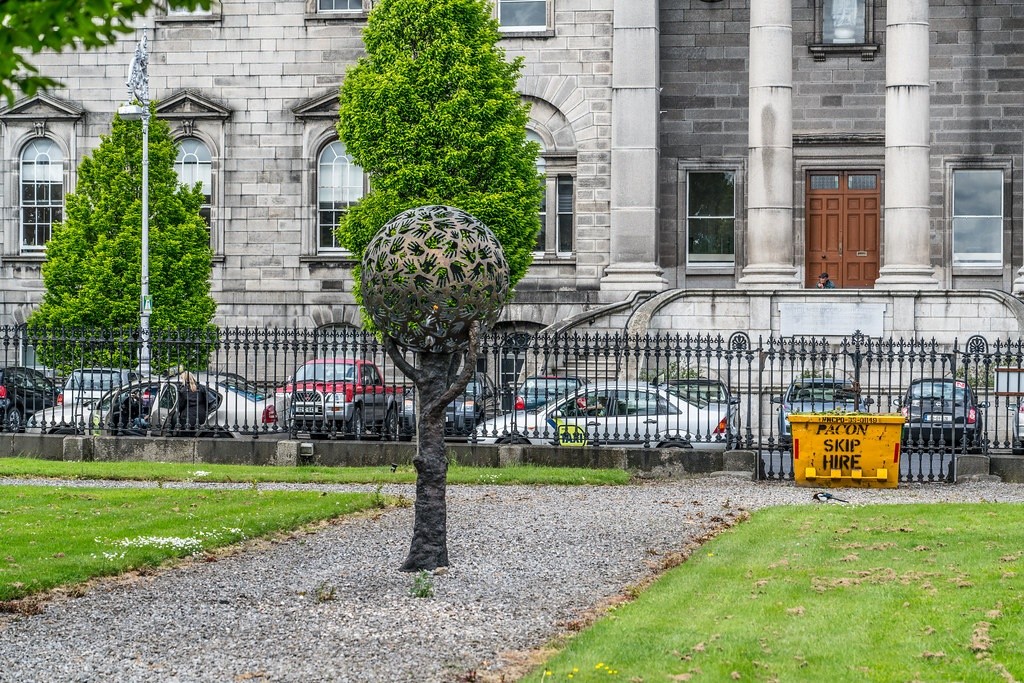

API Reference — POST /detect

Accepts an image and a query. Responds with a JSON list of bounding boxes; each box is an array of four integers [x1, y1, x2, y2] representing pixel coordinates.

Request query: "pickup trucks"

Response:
[[268, 358, 406, 441]]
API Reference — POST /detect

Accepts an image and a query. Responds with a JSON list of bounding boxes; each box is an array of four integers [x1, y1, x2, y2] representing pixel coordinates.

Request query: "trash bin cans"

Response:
[[787, 412, 905, 489]]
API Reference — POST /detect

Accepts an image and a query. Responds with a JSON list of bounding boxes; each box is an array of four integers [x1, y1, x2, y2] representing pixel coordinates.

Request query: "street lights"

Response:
[[117, 29, 154, 373]]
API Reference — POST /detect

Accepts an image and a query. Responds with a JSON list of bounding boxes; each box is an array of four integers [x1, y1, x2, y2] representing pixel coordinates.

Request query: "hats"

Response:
[[819, 273, 828, 279]]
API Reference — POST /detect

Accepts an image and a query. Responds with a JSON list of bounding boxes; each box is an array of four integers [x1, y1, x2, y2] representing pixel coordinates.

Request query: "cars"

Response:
[[893, 378, 991, 454], [24, 371, 295, 438], [467, 378, 739, 451], [396, 373, 500, 442], [0, 366, 61, 434], [1008, 395, 1024, 455], [514, 373, 593, 412], [773, 377, 874, 452], [57, 366, 138, 407], [654, 376, 741, 405]]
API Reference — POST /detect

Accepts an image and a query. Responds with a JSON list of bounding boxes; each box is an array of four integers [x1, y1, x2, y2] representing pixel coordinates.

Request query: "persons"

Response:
[[815, 273, 835, 289], [597, 392, 606, 416], [133, 413, 150, 428], [174, 372, 207, 436]]
